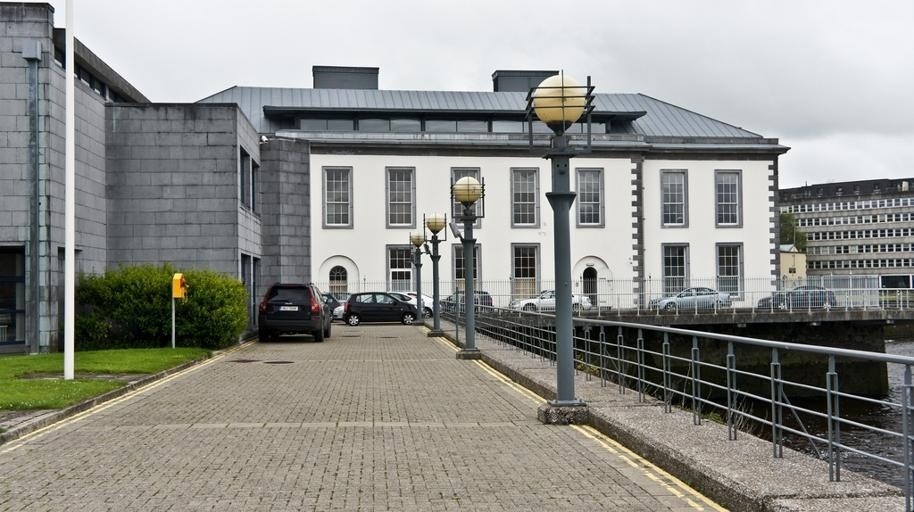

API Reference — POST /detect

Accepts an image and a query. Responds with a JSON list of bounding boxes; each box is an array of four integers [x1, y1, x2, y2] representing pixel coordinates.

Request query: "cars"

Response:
[[758, 285, 835, 309], [508, 290, 593, 312], [259, 282, 441, 343], [441, 291, 493, 312], [649, 287, 732, 312]]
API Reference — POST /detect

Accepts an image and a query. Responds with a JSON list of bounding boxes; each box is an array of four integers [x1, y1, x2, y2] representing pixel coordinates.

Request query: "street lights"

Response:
[[450, 174, 484, 354], [525, 69, 596, 407], [410, 212, 446, 332]]
